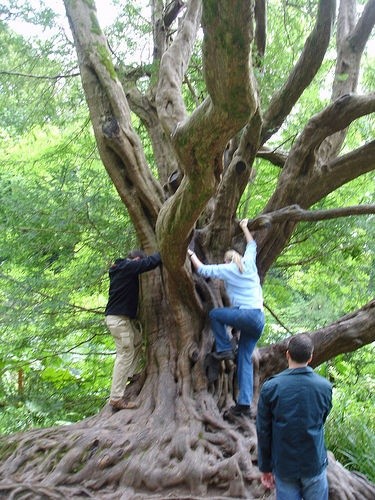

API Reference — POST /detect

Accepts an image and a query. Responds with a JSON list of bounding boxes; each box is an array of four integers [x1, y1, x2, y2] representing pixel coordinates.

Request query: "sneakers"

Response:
[[110, 398, 136, 410], [130, 374, 140, 381], [213, 350, 235, 360], [231, 404, 251, 415]]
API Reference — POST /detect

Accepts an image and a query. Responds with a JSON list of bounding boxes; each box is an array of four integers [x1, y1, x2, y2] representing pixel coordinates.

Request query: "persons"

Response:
[[255, 332, 332, 500], [104, 249, 161, 409], [187, 218, 266, 416]]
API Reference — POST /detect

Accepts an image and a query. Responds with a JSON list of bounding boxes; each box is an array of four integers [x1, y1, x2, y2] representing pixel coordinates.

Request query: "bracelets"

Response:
[[189, 252, 195, 257]]
